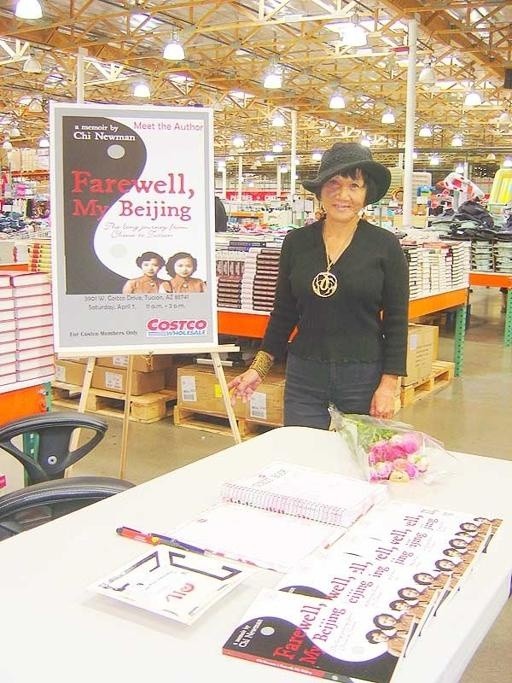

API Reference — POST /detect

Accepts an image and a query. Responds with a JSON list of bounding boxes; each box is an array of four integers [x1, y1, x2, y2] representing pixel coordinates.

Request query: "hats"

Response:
[[300, 140, 393, 205]]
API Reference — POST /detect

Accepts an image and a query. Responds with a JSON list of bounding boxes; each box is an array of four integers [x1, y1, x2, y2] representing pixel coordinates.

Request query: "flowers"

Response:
[[326, 402, 432, 485]]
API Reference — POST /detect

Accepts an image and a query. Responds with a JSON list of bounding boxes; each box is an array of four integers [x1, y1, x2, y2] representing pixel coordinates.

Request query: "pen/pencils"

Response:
[[116, 526, 212, 557]]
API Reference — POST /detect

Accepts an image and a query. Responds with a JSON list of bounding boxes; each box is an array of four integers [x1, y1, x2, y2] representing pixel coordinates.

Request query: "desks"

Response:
[[0, 426, 512, 683]]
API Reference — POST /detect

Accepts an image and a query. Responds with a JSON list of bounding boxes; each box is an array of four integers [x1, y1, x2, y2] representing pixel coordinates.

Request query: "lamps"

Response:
[[15, 0, 481, 144]]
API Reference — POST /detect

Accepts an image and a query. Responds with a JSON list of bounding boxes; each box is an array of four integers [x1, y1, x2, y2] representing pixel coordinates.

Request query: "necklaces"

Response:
[[309, 218, 361, 298]]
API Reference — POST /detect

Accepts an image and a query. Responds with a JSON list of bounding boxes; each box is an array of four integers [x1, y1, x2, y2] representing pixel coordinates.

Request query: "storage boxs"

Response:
[[397, 322, 439, 388], [174, 362, 287, 425], [57, 354, 197, 397]]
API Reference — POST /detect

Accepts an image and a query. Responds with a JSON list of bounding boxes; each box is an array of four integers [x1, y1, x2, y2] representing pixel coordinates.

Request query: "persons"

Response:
[[122, 251, 173, 293], [159, 252, 207, 293], [215, 195, 227, 232], [227, 140, 411, 430]]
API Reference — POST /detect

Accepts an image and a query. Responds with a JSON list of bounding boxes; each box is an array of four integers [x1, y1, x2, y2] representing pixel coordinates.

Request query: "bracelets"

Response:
[[249, 350, 275, 382]]
